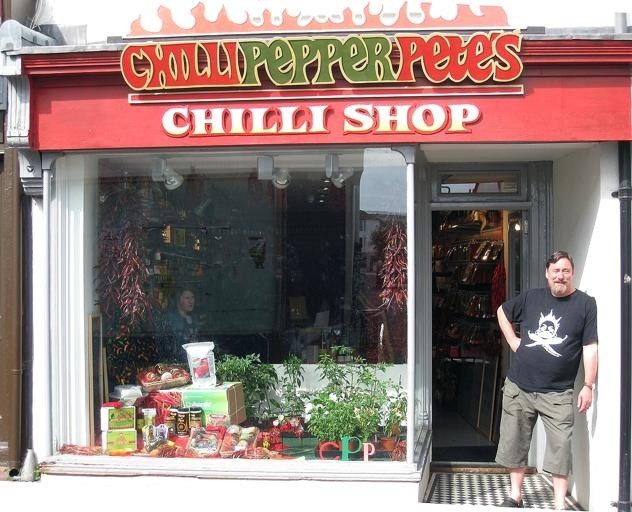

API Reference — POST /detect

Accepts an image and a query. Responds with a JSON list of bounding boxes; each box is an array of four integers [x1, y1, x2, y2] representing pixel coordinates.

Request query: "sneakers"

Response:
[[500, 496, 523, 507]]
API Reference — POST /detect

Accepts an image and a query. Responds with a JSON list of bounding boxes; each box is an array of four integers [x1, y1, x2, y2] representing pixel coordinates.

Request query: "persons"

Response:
[[168, 283, 200, 339], [493, 251, 601, 511], [298, 288, 362, 353]]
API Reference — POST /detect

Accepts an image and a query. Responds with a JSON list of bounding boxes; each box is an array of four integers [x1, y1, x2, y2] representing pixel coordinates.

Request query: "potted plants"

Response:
[[305, 342, 421, 455]]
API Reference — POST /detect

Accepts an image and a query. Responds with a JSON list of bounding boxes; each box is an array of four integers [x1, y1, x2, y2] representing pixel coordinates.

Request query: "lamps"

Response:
[[257, 153, 291, 189], [148, 156, 185, 192], [321, 154, 354, 190]]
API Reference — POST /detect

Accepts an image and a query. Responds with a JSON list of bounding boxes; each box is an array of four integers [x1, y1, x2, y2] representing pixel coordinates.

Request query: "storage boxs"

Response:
[[173, 380, 247, 427], [100, 428, 141, 456], [99, 404, 138, 432]]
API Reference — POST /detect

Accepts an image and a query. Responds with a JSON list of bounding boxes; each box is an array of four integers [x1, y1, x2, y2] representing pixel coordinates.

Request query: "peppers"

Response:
[[93, 187, 151, 327], [376, 216, 407, 316]]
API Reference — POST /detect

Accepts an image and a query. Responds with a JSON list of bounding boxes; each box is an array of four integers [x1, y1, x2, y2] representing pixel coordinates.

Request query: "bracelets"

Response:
[[583, 381, 593, 391]]
